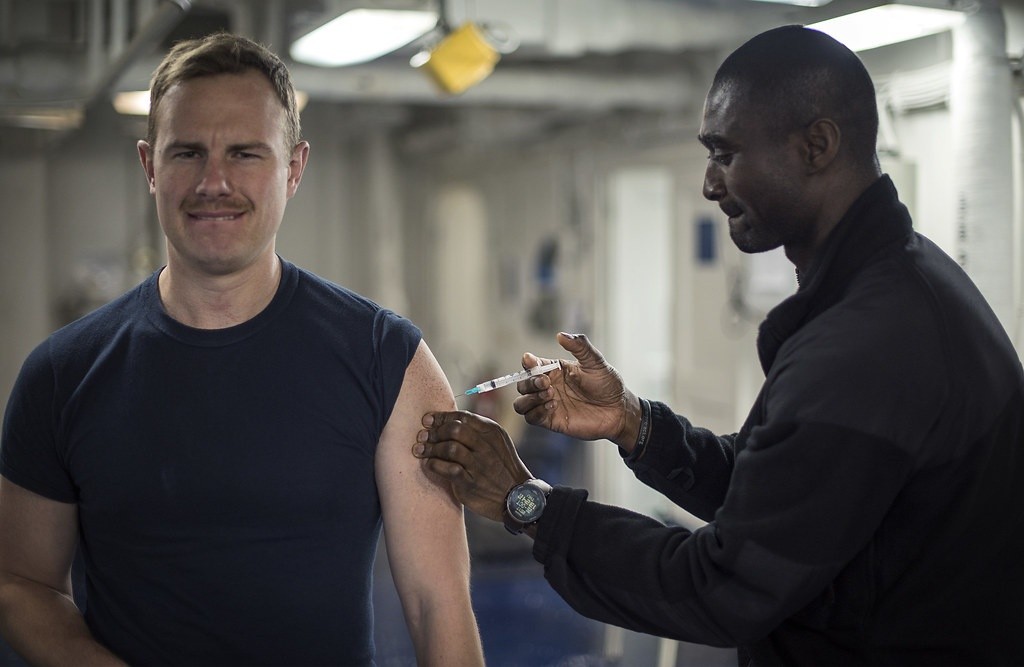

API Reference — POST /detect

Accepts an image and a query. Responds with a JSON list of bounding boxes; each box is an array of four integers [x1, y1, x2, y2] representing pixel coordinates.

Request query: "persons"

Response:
[[410, 24, 1024, 667], [0, 32, 491, 667]]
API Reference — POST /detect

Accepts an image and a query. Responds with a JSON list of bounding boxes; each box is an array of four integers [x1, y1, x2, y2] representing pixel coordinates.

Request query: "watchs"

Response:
[[501, 477, 553, 536]]
